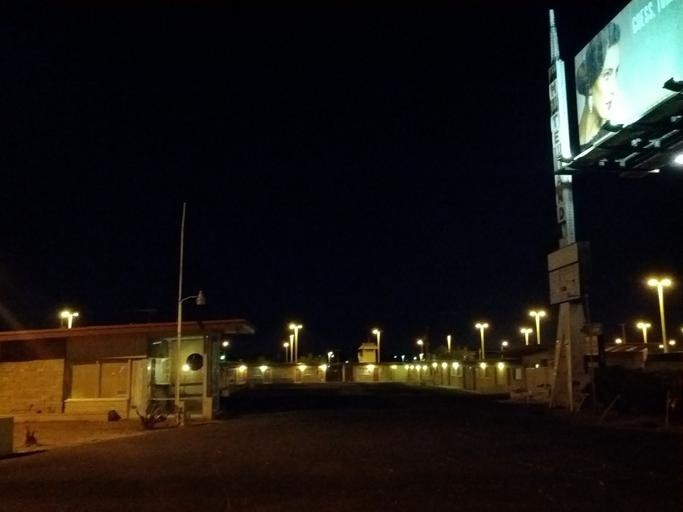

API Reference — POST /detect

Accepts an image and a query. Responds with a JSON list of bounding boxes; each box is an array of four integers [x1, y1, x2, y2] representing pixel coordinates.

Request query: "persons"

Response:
[[576, 23, 626, 153]]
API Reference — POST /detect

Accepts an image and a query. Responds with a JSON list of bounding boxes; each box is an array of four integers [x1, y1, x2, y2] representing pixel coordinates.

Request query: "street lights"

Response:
[[530, 310, 546, 344], [283, 342, 289, 363], [58, 309, 80, 329], [372, 329, 381, 364], [520, 328, 533, 346], [646, 277, 674, 353], [636, 321, 652, 343], [446, 334, 452, 353], [289, 323, 303, 363], [474, 322, 489, 359], [416, 338, 424, 354]]
